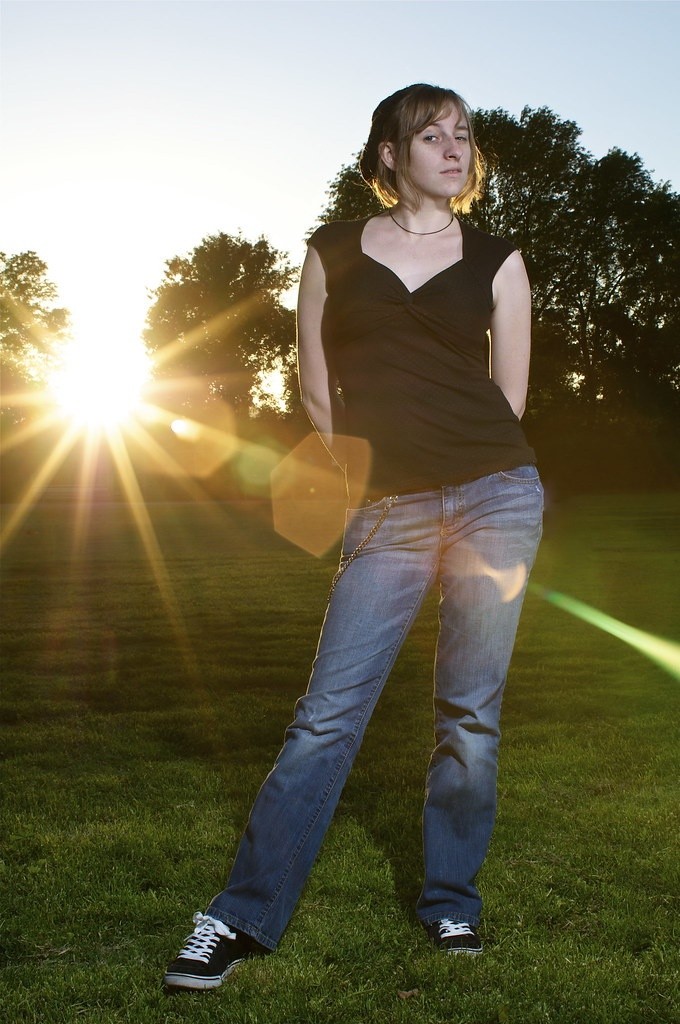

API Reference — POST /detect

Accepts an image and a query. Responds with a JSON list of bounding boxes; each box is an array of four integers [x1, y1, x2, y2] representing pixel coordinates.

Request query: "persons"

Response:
[[163, 83, 546, 992]]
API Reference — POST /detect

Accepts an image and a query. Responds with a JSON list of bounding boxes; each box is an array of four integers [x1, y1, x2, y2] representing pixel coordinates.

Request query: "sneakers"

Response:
[[427, 918, 484, 956], [164, 909, 251, 990]]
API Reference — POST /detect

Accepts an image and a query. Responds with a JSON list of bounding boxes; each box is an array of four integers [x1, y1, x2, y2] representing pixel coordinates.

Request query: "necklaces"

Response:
[[388, 207, 454, 235]]
